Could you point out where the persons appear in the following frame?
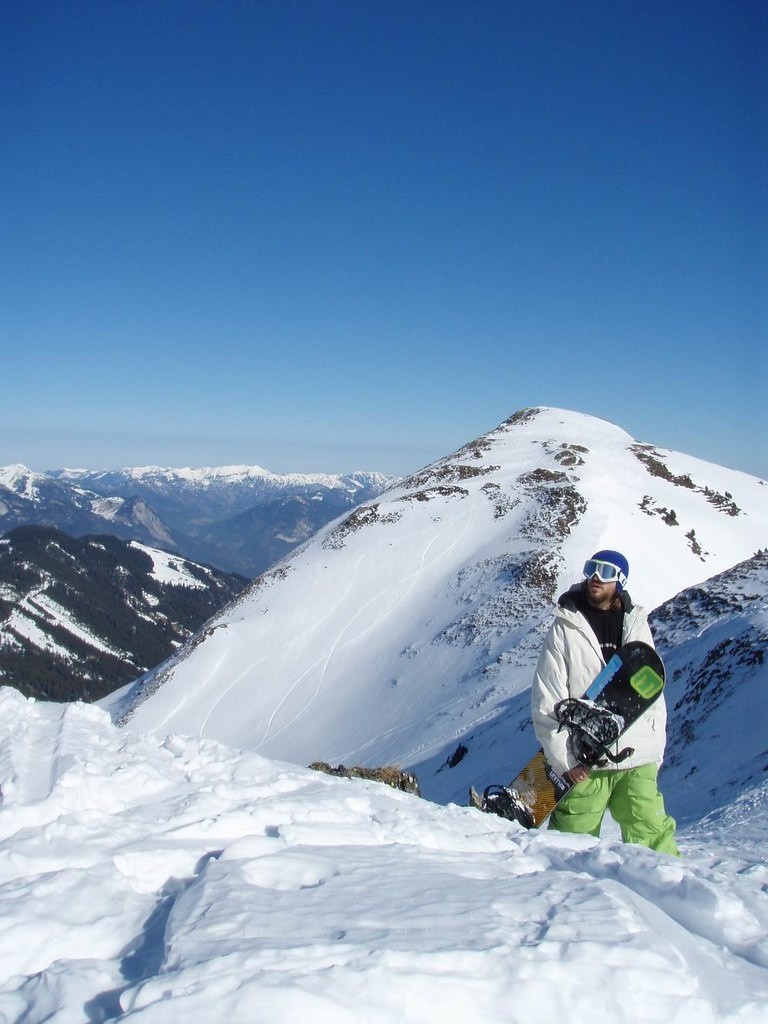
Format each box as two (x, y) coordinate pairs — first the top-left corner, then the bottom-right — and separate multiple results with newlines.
(530, 550), (681, 858)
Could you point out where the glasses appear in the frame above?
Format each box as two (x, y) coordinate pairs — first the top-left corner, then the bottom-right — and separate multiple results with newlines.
(582, 560), (620, 584)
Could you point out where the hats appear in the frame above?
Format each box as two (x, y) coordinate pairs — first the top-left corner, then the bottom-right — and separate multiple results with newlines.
(582, 549), (629, 594)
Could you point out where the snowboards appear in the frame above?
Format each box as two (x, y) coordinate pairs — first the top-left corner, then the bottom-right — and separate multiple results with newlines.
(485, 641), (666, 827)
